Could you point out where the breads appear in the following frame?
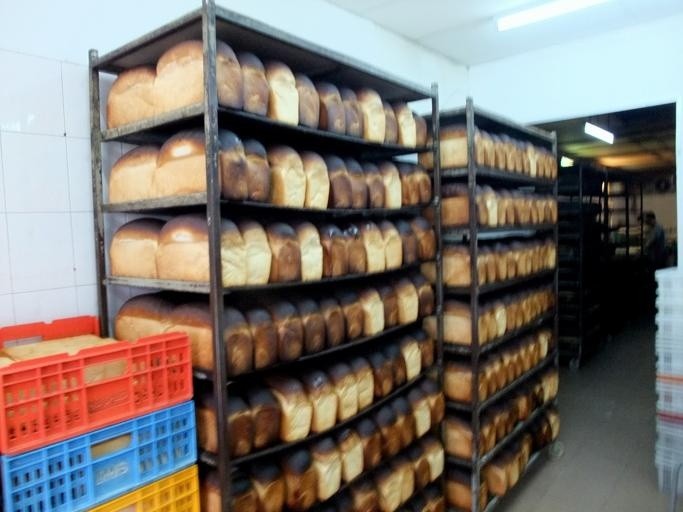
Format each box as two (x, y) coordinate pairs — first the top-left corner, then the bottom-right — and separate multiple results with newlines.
(0, 39), (561, 511)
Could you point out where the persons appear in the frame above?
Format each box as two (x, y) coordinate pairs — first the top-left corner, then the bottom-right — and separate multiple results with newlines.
(636, 208), (665, 261)
